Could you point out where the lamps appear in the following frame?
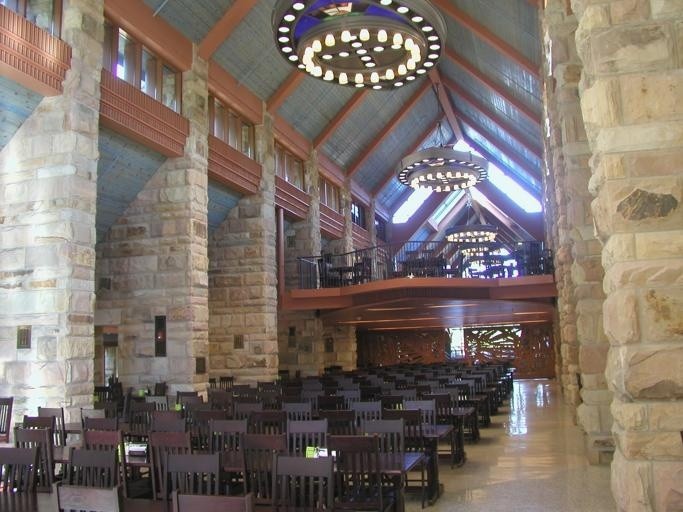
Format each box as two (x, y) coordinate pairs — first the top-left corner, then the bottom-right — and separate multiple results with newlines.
(447, 226), (496, 246)
(272, 1), (445, 93)
(393, 147), (488, 194)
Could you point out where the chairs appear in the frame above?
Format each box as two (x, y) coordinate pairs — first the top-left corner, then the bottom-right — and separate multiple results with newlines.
(319, 244), (550, 288)
